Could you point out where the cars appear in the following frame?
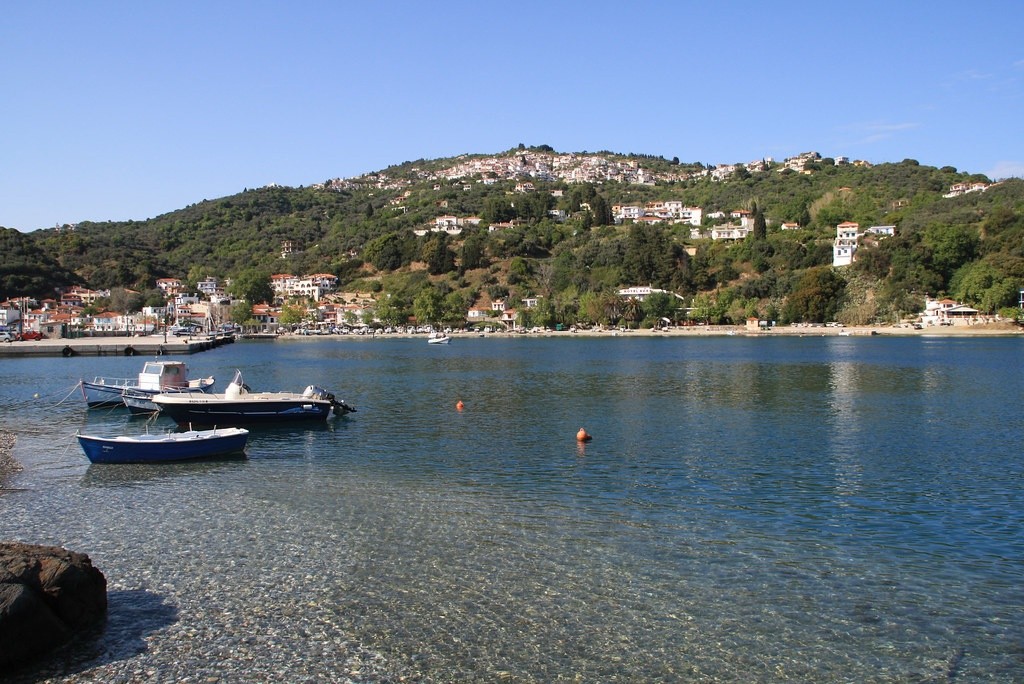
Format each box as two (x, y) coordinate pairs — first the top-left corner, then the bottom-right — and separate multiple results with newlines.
(16, 330), (45, 341)
(0, 332), (16, 343)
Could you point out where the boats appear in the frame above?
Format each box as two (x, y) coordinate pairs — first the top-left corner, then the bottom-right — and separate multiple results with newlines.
(150, 367), (358, 428)
(76, 428), (249, 464)
(427, 333), (450, 344)
(120, 359), (216, 416)
(79, 374), (141, 411)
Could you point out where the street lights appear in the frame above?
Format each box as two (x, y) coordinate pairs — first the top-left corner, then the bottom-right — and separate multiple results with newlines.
(164, 294), (168, 343)
